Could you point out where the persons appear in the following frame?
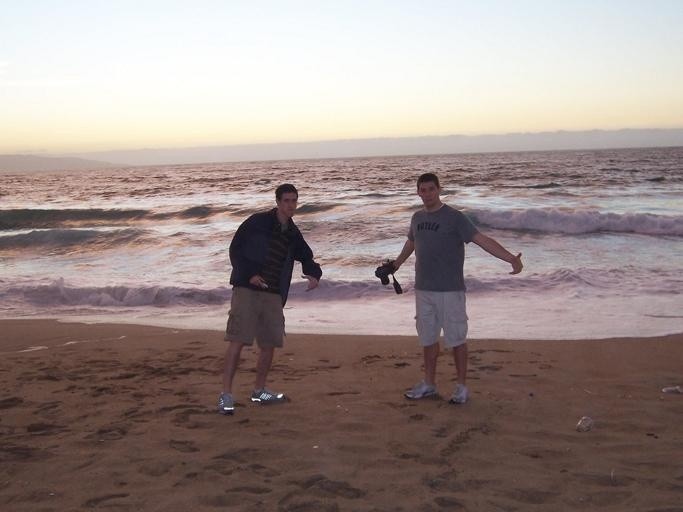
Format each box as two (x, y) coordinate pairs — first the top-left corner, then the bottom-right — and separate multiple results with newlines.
(218, 183), (322, 415)
(376, 173), (523, 404)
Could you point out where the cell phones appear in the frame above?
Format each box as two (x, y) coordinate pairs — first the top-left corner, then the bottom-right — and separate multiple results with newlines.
(263, 282), (269, 289)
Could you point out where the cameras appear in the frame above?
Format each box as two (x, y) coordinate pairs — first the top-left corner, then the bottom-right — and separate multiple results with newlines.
(375, 264), (396, 285)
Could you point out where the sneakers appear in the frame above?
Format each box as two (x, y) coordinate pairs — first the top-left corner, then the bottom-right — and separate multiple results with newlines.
(448, 383), (467, 403)
(251, 387), (284, 401)
(217, 392), (234, 414)
(404, 380), (437, 398)
